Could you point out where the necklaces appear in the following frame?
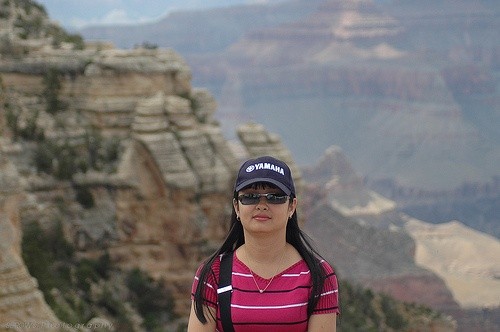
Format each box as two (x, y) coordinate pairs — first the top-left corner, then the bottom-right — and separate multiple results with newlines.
(245, 249), (283, 293)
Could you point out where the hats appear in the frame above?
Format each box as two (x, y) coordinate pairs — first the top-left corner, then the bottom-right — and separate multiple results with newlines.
(236, 156), (295, 195)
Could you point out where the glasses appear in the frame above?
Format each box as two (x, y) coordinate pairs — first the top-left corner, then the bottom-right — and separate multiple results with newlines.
(239, 192), (287, 204)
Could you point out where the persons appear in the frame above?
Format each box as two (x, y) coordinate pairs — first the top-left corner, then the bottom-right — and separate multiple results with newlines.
(186, 157), (342, 332)
